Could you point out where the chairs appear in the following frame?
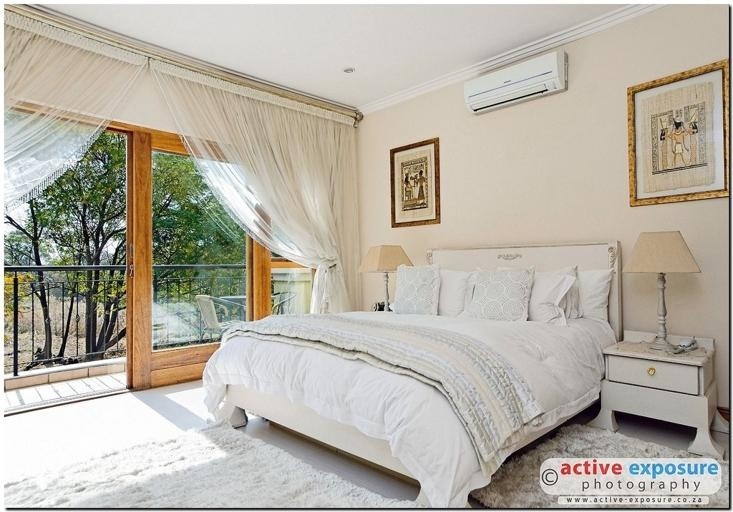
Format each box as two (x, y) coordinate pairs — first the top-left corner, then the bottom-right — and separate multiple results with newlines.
(195, 294), (247, 340)
(270, 290), (300, 315)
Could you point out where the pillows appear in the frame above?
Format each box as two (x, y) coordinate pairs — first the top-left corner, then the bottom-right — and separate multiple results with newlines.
(389, 263), (614, 326)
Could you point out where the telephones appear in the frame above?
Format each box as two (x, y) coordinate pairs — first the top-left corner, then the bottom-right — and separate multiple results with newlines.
(679, 338), (697, 351)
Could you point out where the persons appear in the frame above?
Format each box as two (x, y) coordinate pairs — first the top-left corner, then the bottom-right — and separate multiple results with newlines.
(660, 118), (698, 169)
(403, 170), (425, 207)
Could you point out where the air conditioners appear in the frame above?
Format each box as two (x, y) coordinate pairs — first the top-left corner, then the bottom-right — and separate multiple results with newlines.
(461, 50), (570, 116)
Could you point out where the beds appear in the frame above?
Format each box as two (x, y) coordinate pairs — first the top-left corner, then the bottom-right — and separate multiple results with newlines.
(197, 238), (628, 509)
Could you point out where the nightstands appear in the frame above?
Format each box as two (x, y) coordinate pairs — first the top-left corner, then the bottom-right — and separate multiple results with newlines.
(584, 328), (730, 460)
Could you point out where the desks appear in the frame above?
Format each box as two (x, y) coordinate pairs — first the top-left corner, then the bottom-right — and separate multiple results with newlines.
(219, 294), (277, 322)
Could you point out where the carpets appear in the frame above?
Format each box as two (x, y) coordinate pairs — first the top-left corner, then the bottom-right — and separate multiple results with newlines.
(5, 411), (428, 508)
(470, 419), (728, 510)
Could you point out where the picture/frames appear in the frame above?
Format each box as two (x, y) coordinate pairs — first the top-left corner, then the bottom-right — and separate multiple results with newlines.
(623, 58), (729, 209)
(389, 135), (440, 230)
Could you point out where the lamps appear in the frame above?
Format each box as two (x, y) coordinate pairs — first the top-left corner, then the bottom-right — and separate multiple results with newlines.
(620, 229), (701, 353)
(355, 243), (413, 312)
